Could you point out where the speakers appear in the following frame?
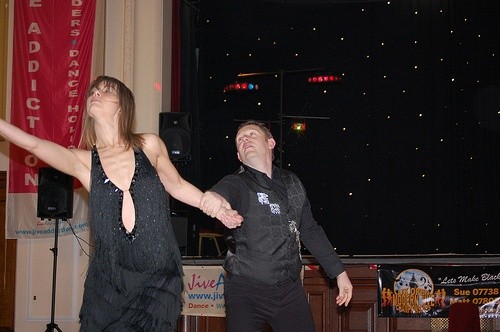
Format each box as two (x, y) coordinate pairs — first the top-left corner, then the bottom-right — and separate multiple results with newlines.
(37, 167), (73, 221)
(159, 112), (193, 165)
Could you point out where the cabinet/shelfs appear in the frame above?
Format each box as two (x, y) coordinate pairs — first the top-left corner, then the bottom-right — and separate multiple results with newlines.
(184, 264), (431, 332)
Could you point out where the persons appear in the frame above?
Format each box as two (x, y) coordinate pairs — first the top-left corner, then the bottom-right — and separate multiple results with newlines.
(0, 75), (244, 332)
(200, 120), (353, 332)
